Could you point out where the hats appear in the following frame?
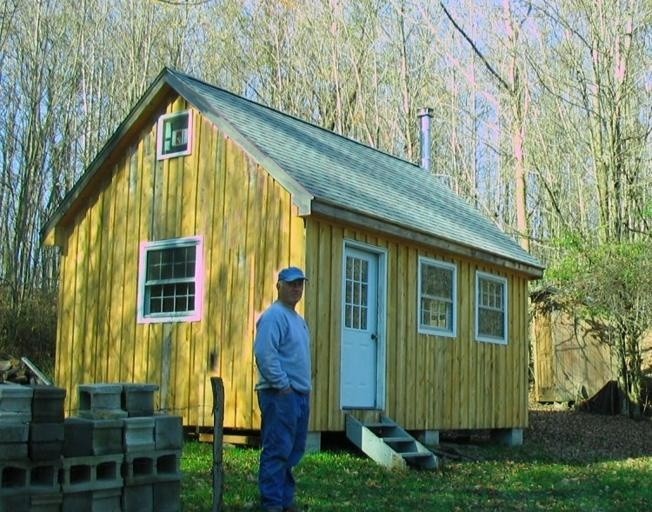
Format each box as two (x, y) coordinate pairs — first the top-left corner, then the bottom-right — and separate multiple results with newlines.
(277, 267), (309, 284)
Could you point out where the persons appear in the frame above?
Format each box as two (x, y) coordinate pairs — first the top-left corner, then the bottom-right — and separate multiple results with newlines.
(250, 266), (313, 509)
